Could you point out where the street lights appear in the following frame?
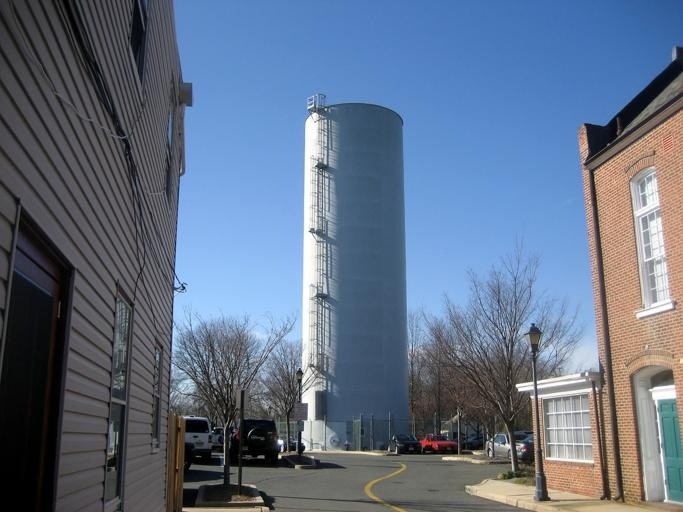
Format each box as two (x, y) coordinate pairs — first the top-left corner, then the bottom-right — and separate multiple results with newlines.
(524, 322), (553, 502)
(454, 399), (465, 455)
(296, 367), (305, 456)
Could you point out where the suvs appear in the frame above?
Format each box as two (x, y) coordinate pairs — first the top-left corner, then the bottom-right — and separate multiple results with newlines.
(230, 417), (278, 465)
(211, 426), (228, 452)
(183, 415), (213, 464)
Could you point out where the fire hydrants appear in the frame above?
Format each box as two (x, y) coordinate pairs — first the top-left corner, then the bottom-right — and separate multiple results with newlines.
(344, 441), (351, 451)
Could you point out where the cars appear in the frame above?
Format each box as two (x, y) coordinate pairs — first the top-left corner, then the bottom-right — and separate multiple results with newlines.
(277, 434), (305, 453)
(385, 433), (421, 455)
(419, 433), (458, 453)
(463, 432), (491, 450)
(486, 431), (528, 461)
